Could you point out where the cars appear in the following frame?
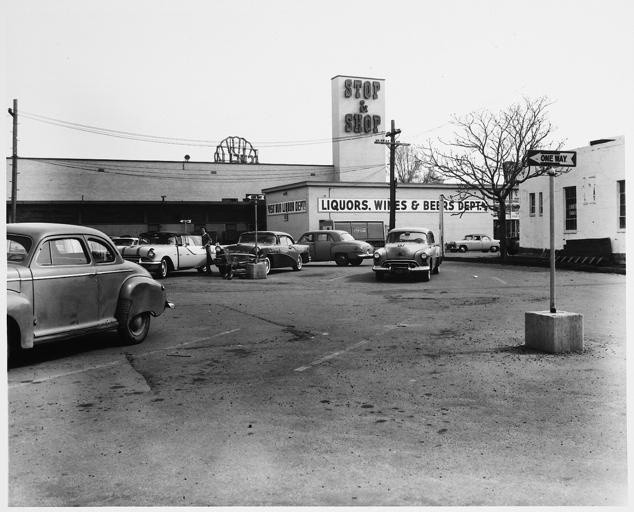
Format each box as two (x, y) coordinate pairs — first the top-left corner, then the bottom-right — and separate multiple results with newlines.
(372, 227), (441, 279)
(213, 231), (312, 276)
(108, 231), (231, 279)
(294, 230), (375, 267)
(7, 219), (176, 367)
(446, 234), (503, 254)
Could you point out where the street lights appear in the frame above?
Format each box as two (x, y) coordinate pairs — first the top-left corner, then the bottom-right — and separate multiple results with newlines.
(247, 194), (265, 264)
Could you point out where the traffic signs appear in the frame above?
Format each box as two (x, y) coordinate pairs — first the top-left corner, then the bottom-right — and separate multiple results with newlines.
(527, 151), (579, 168)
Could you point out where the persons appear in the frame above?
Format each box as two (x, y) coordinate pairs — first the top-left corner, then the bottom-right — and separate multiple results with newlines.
(215, 245), (234, 279)
(200, 227), (213, 274)
